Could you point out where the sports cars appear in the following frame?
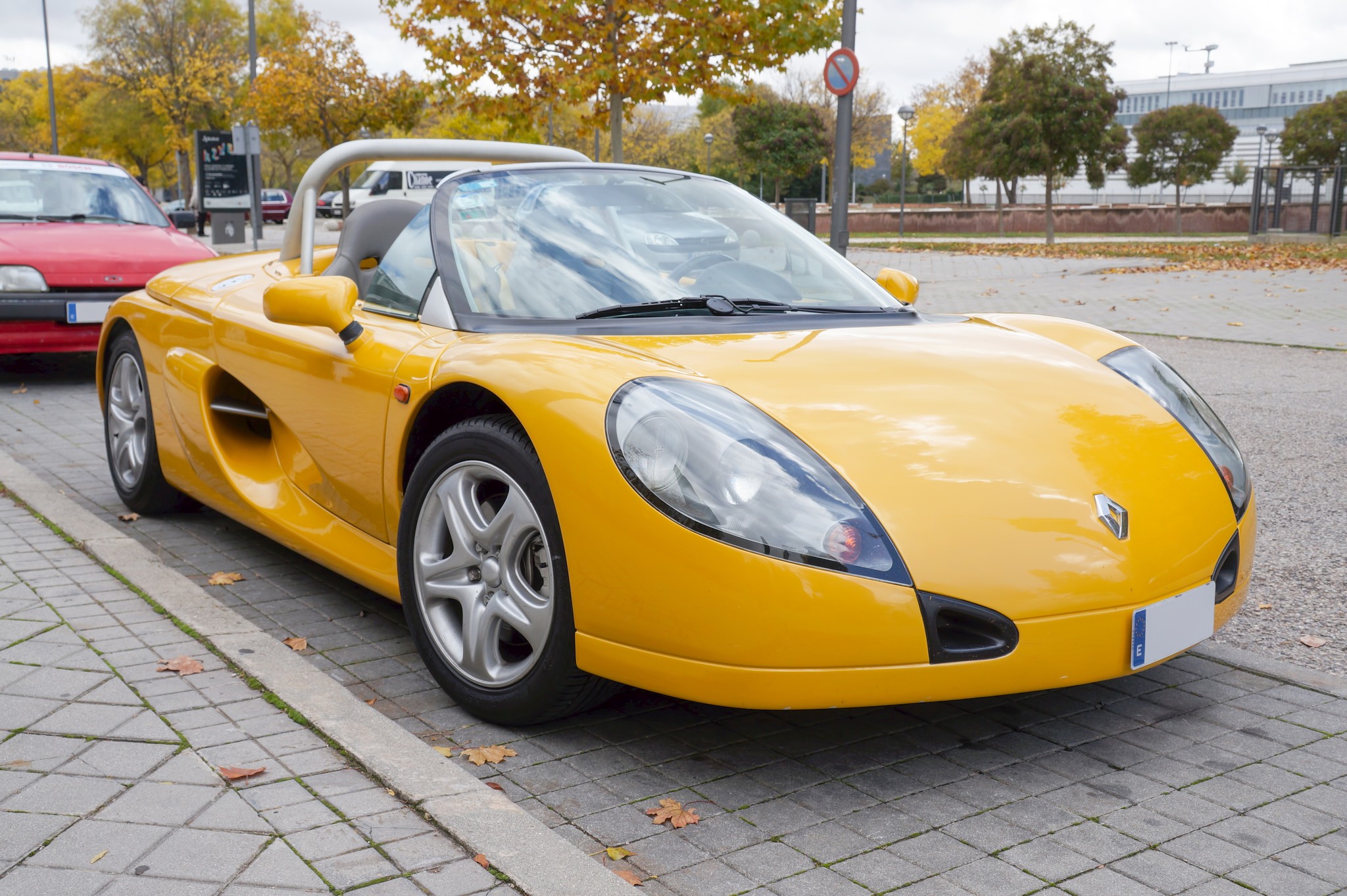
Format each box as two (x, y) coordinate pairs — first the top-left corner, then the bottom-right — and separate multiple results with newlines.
(96, 138), (1257, 726)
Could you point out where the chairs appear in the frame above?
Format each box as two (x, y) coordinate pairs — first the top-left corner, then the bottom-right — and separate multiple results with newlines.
(509, 186), (633, 319)
(321, 197), (510, 319)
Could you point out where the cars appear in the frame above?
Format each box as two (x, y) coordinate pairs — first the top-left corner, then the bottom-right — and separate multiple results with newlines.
(316, 190), (344, 217)
(0, 150), (221, 362)
(207, 187), (294, 225)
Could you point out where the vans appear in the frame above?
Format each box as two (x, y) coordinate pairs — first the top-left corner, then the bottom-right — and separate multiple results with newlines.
(332, 161), (491, 218)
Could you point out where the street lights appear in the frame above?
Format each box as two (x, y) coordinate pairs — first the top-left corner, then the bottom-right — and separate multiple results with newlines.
(1264, 130), (1281, 207)
(703, 133), (714, 175)
(1257, 124), (1268, 204)
(898, 103), (914, 235)
